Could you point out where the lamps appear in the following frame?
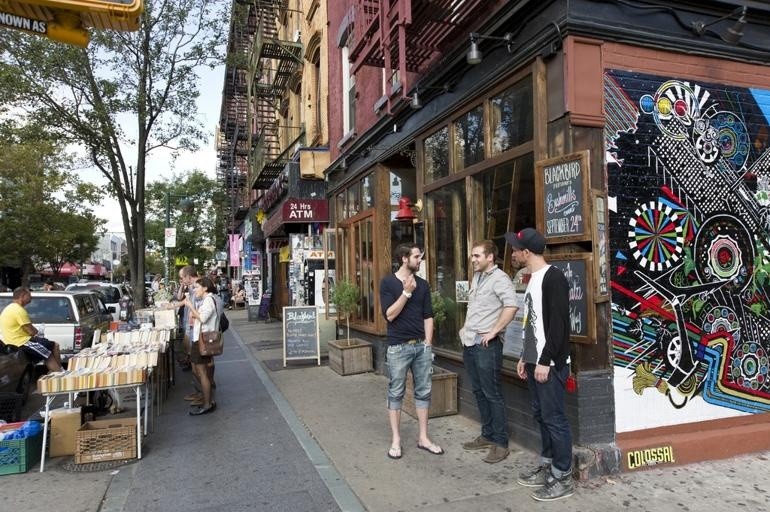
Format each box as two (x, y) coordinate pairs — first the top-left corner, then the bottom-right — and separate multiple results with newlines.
(690, 3), (750, 47)
(322, 169), (338, 183)
(338, 151), (365, 169)
(465, 31), (518, 66)
(407, 78), (456, 111)
(394, 196), (424, 219)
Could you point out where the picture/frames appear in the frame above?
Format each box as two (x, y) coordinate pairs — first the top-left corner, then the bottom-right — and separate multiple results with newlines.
(587, 188), (610, 304)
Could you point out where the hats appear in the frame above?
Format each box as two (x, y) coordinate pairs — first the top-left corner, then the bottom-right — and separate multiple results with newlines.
(503, 227), (546, 255)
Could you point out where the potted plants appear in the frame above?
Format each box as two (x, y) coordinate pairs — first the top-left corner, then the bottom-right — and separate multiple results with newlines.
(325, 277), (375, 376)
(401, 281), (460, 421)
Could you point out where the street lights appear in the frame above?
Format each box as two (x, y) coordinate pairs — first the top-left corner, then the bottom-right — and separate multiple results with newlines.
(162, 190), (198, 294)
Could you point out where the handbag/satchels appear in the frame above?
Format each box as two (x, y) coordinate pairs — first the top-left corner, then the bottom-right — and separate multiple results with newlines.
(198, 330), (225, 358)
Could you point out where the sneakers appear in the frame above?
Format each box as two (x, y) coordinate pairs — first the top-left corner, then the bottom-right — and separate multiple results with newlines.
(182, 392), (198, 401)
(482, 445), (511, 464)
(186, 403), (214, 416)
(514, 460), (553, 488)
(462, 434), (494, 450)
(188, 394), (205, 406)
(529, 465), (576, 502)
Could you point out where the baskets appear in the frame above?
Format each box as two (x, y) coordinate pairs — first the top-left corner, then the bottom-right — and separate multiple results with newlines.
(73, 417), (144, 464)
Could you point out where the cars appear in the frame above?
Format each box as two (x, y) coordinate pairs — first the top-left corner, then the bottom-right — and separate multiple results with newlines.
(0, 280), (153, 377)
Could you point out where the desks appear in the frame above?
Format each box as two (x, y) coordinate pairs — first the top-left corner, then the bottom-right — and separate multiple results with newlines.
(36, 331), (175, 474)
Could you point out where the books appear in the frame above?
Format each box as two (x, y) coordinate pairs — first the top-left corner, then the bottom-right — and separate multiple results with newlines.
(35, 304), (177, 394)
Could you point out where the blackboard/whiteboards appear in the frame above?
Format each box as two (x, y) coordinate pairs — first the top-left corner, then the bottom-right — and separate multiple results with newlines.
(283, 305), (321, 359)
(589, 188), (613, 304)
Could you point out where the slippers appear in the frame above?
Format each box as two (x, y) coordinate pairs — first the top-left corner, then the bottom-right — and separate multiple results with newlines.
(387, 442), (404, 460)
(415, 440), (444, 456)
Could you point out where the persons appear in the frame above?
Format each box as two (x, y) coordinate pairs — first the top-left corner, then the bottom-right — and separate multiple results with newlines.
(503, 228), (577, 503)
(0, 285), (61, 372)
(458, 239), (520, 462)
(380, 242), (443, 459)
(173, 264), (216, 406)
(184, 277), (223, 416)
(151, 274), (162, 292)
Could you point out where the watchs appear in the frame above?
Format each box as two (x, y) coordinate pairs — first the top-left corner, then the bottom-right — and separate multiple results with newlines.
(402, 290), (412, 298)
